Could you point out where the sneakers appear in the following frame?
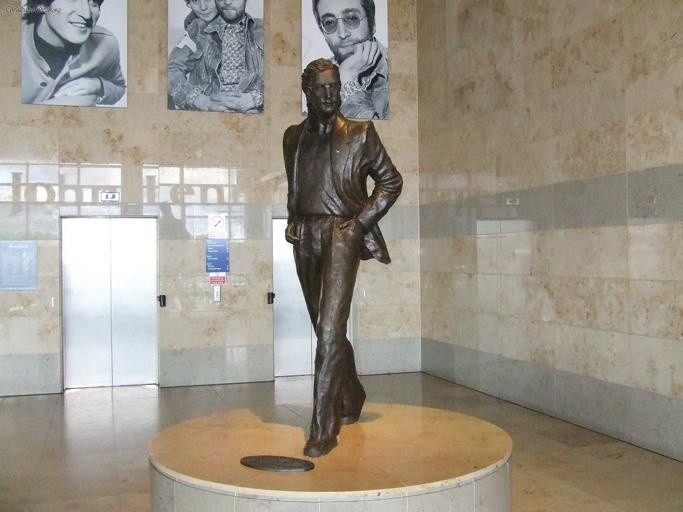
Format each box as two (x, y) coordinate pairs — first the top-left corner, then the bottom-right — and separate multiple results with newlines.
(302, 436), (338, 459)
(343, 386), (366, 426)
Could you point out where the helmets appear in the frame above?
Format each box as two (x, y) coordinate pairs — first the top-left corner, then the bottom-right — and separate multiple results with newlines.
(320, 15), (361, 34)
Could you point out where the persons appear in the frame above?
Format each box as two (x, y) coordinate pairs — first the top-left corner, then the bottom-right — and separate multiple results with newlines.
(167, 0), (264, 113)
(282, 57), (404, 454)
(183, 0), (219, 30)
(301, 0), (389, 118)
(19, 0), (127, 106)
